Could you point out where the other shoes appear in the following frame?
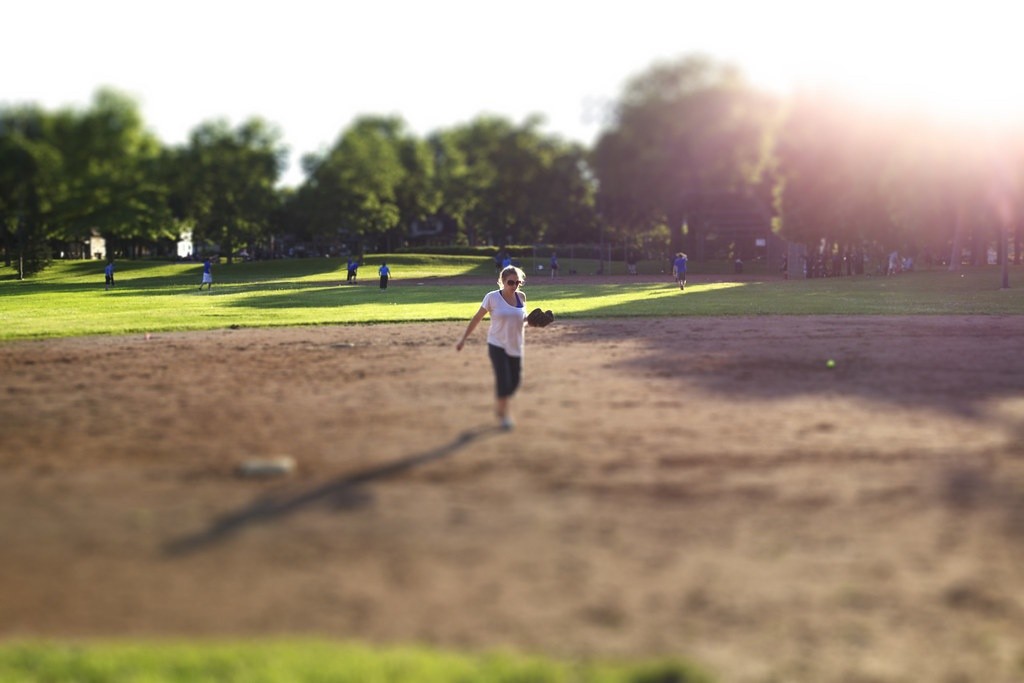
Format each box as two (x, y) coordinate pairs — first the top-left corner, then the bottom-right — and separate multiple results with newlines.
(500, 418), (513, 428)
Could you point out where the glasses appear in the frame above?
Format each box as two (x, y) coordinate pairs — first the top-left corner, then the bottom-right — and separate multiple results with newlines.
(506, 280), (521, 286)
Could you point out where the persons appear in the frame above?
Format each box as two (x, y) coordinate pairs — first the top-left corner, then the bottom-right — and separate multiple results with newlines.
(673, 252), (687, 291)
(199, 256), (212, 291)
(456, 265), (554, 429)
(104, 258), (115, 291)
(379, 261), (391, 290)
(492, 251), (561, 283)
(626, 250), (637, 275)
(345, 254), (360, 285)
(781, 240), (916, 279)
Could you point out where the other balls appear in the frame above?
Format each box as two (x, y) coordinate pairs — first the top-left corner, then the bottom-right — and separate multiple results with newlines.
(826, 358), (837, 370)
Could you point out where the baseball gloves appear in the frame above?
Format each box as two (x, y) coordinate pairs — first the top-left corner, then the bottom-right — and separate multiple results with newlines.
(527, 307), (553, 328)
